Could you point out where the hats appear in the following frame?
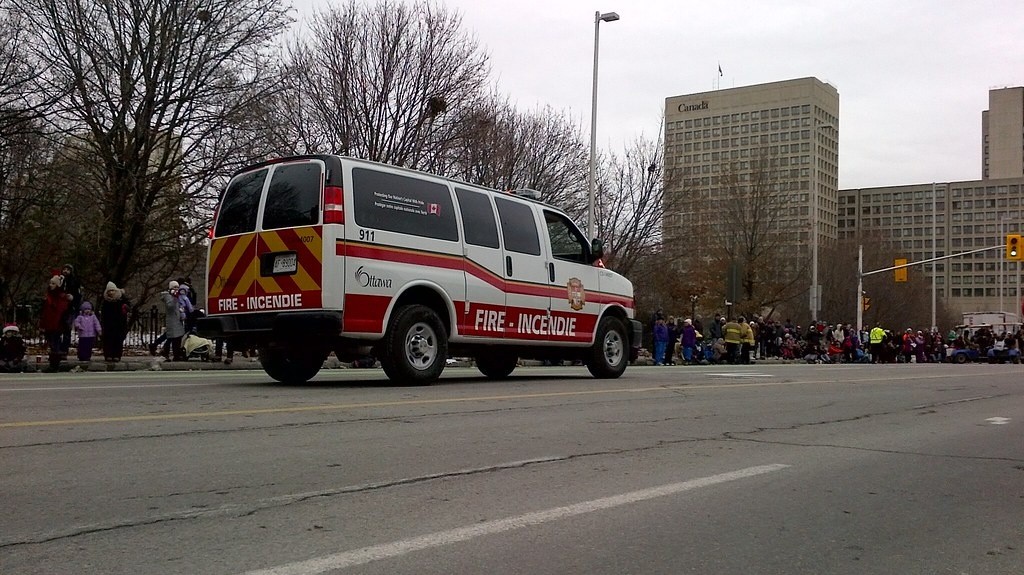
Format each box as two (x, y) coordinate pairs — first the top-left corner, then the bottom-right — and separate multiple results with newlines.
(51, 275), (65, 287)
(684, 319), (692, 325)
(104, 281), (118, 295)
(81, 302), (92, 311)
(169, 281), (179, 289)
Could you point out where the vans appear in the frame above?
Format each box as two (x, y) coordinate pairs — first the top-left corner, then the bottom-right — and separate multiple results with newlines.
(196, 152), (643, 387)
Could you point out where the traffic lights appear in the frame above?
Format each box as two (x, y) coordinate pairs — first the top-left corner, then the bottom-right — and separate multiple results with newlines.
(864, 298), (870, 311)
(1006, 235), (1022, 260)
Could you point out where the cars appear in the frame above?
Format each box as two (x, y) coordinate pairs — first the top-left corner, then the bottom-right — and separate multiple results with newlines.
(944, 329), (1023, 365)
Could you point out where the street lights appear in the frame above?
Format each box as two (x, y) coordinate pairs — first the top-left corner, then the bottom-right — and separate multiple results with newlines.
(587, 10), (621, 244)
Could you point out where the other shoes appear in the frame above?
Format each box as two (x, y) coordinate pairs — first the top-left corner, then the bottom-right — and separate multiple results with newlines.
(224, 358), (233, 365)
(671, 363), (674, 366)
(148, 343), (157, 356)
(666, 363), (669, 365)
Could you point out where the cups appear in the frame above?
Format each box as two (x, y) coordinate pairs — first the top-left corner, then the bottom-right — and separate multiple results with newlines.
(36, 355), (41, 363)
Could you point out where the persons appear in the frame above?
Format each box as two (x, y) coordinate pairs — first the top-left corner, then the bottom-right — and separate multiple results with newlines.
(40, 263), (80, 366)
(148, 276), (198, 362)
(648, 308), (1024, 366)
(72, 302), (103, 363)
(0, 322), (26, 368)
(99, 280), (132, 363)
(211, 338), (235, 365)
(240, 342), (260, 357)
